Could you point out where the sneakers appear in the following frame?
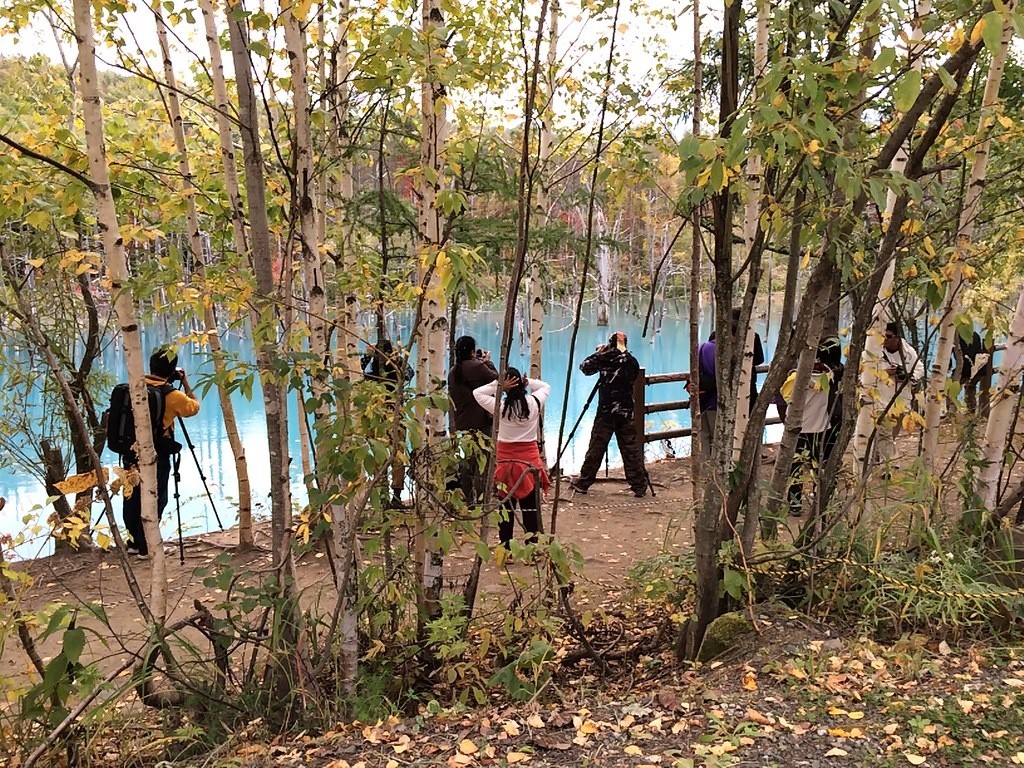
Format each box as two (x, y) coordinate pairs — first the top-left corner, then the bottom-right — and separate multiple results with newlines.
(893, 463), (901, 470)
(570, 480), (589, 495)
(879, 467), (891, 481)
(504, 553), (514, 565)
(789, 506), (801, 516)
(631, 487), (647, 497)
(126, 545), (149, 559)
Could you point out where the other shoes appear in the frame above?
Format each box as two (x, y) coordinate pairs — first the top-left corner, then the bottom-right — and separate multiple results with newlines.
(381, 500), (411, 510)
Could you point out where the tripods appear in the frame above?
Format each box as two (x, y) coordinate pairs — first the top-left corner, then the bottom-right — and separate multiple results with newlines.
(549, 378), (656, 496)
(90, 416), (225, 565)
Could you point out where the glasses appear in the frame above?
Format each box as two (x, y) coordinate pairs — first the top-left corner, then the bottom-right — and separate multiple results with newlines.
(884, 334), (897, 339)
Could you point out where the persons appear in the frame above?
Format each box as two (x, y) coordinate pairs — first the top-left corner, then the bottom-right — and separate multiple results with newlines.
(122, 348), (200, 560)
(570, 332), (647, 498)
(698, 307), (764, 483)
(776, 328), (844, 517)
(961, 331), (980, 416)
(448, 336), (499, 511)
(877, 322), (925, 480)
(473, 367), (551, 565)
(361, 339), (414, 511)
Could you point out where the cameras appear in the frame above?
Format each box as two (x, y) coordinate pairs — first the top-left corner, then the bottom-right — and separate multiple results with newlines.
(476, 350), (487, 361)
(168, 368), (182, 383)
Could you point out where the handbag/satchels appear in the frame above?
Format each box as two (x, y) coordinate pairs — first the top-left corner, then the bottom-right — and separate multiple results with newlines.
(537, 440), (544, 454)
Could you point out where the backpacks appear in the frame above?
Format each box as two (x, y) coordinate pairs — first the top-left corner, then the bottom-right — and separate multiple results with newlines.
(108, 383), (166, 455)
